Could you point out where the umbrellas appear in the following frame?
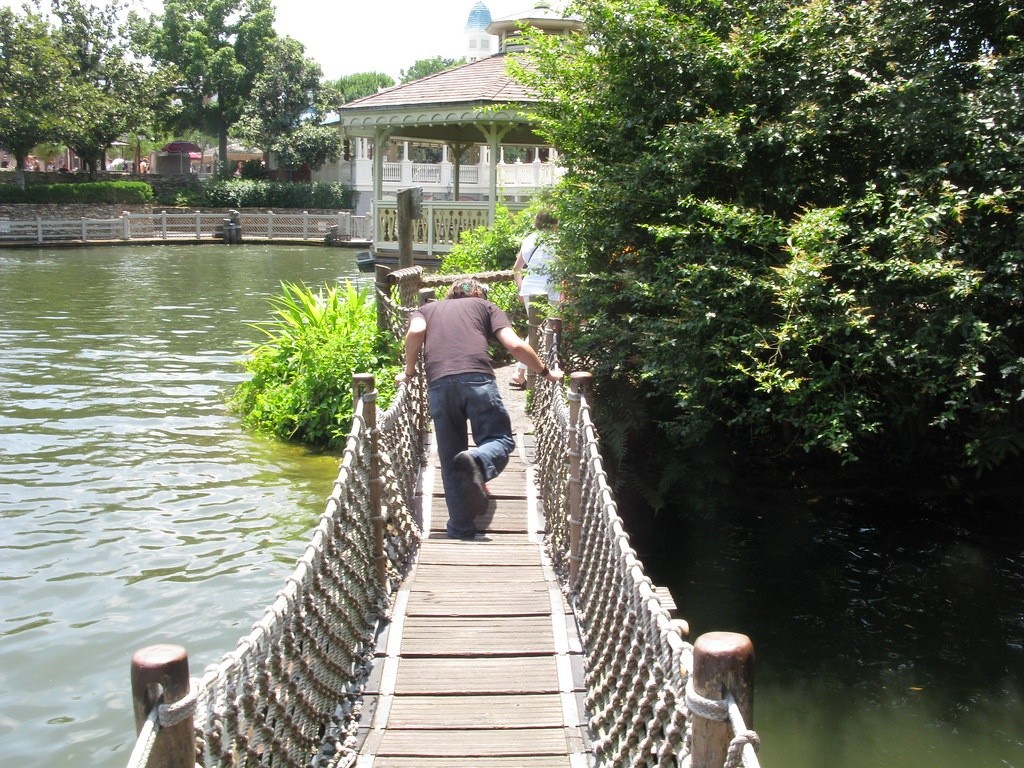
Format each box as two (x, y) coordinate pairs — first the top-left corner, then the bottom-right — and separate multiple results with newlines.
(162, 140), (202, 174)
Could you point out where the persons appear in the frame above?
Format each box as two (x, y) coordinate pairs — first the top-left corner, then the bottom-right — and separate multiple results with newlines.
(510, 208), (566, 391)
(1, 156), (149, 175)
(393, 275), (567, 540)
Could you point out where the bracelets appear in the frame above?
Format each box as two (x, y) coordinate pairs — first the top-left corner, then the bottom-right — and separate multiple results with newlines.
(405, 370), (416, 378)
(536, 366), (550, 380)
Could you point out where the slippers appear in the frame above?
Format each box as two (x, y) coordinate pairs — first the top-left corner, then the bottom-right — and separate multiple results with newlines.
(509, 380), (528, 390)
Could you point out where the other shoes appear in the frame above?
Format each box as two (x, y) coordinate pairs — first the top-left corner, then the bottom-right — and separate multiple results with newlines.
(454, 451), (487, 515)
(448, 526), (476, 540)
